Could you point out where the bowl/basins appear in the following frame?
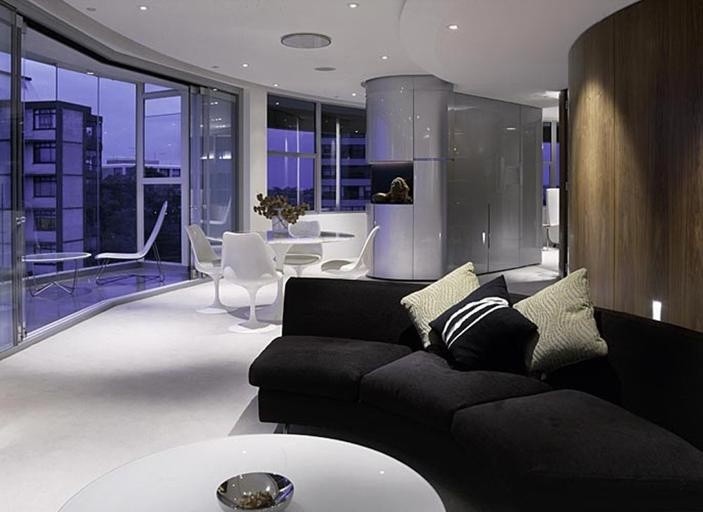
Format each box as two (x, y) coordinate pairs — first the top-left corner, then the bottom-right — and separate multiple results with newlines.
(216, 472), (293, 511)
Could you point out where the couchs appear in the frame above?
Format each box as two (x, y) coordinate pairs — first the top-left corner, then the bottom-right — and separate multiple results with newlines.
(246, 278), (703, 512)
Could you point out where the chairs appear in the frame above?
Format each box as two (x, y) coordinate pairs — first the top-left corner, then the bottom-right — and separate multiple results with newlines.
(217, 231), (281, 329)
(543, 188), (560, 246)
(92, 199), (170, 281)
(183, 222), (239, 315)
(320, 224), (381, 279)
(273, 221), (322, 276)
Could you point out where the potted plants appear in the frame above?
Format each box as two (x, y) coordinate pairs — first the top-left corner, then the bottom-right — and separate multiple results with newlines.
(253, 189), (309, 239)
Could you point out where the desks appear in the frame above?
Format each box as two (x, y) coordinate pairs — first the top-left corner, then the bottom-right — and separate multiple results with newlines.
(219, 229), (355, 321)
(21, 250), (92, 297)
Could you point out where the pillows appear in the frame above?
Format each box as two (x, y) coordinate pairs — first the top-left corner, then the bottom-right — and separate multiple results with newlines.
(399, 261), (481, 349)
(427, 273), (541, 371)
(510, 266), (609, 380)
